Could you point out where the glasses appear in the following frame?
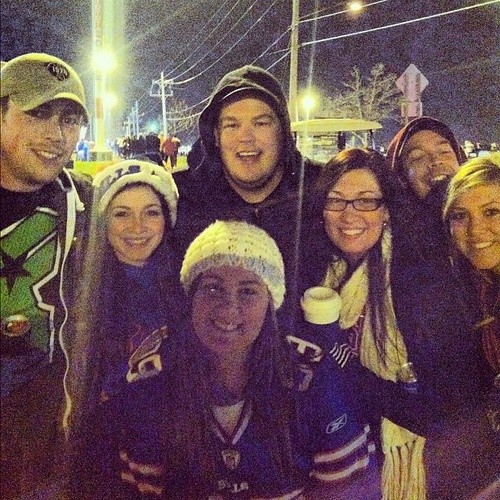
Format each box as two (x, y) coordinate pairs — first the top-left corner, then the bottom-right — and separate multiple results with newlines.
(324, 195), (386, 213)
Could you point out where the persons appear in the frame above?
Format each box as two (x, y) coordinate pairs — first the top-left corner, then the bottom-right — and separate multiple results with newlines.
(157, 64), (335, 338)
(114, 133), (181, 168)
(1, 51), (96, 493)
(282, 146), (481, 500)
(74, 155), (187, 417)
(385, 114), (468, 202)
(441, 152), (500, 498)
(82, 216), (381, 500)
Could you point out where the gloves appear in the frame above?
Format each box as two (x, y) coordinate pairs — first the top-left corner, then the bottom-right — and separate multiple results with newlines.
(298, 322), (353, 373)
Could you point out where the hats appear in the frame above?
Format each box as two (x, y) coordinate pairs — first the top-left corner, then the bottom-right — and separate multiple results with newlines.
(180, 221), (286, 309)
(91, 154), (180, 228)
(0, 51), (90, 125)
